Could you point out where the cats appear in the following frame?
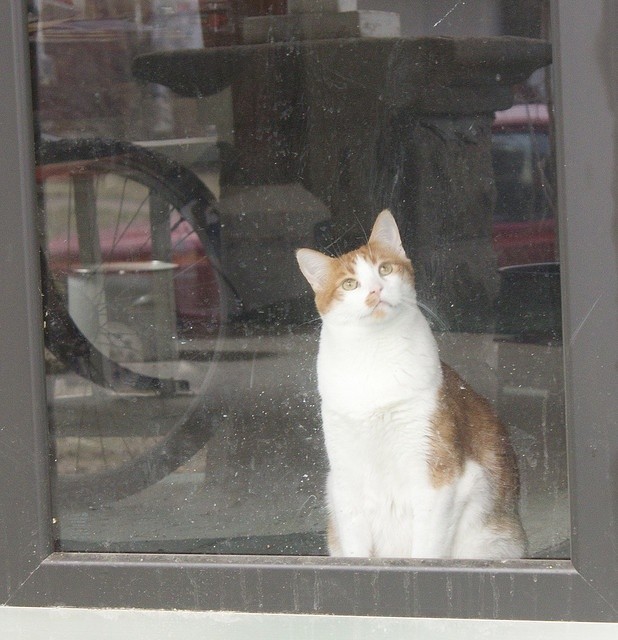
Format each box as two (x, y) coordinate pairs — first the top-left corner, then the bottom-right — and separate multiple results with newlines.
(297, 208), (528, 560)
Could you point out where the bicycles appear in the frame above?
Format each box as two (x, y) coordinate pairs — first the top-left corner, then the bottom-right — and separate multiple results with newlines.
(37, 137), (244, 507)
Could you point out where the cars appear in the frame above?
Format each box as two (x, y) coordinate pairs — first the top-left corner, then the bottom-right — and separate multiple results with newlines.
(44, 103), (562, 340)
